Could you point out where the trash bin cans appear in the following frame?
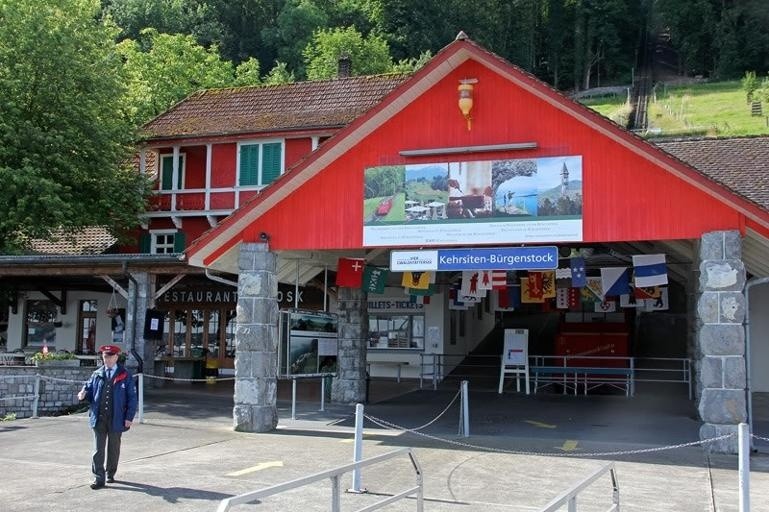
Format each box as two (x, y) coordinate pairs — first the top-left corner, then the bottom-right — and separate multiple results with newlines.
(206, 359), (218, 385)
(194, 360), (205, 384)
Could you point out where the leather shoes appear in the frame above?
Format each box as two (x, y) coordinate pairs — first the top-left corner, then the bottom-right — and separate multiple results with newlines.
(90, 484), (104, 489)
(106, 471), (114, 483)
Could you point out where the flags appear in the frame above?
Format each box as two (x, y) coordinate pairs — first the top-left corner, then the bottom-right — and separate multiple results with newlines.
(336, 253), (669, 313)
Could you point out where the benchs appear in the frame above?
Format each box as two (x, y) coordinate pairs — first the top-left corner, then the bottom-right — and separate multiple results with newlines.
(367, 360), (409, 384)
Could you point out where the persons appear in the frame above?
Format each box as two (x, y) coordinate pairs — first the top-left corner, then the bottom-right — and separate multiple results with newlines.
(76, 345), (138, 490)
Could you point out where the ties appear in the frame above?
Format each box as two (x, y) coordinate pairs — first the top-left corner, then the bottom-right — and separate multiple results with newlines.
(107, 369), (112, 378)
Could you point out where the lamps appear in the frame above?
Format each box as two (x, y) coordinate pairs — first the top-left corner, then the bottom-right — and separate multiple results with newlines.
(457, 79), (479, 132)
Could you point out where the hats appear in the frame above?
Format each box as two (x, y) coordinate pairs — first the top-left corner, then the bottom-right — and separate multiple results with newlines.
(99, 345), (122, 355)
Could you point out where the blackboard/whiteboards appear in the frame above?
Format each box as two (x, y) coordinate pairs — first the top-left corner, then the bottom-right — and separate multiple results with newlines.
(143, 308), (163, 340)
(505, 332), (526, 365)
(112, 308), (125, 333)
